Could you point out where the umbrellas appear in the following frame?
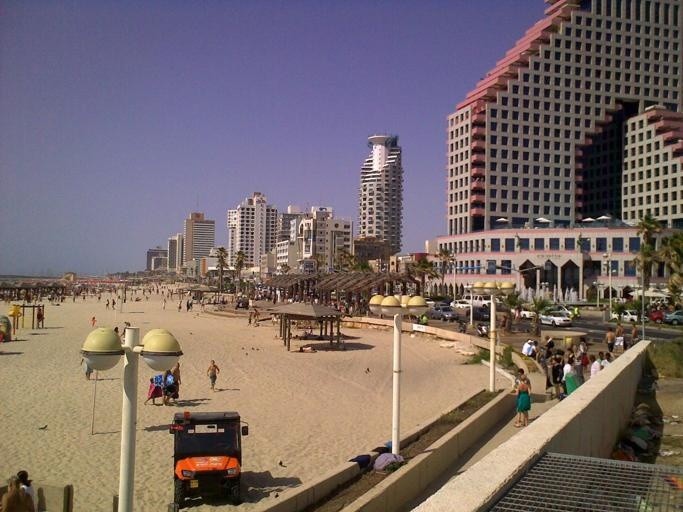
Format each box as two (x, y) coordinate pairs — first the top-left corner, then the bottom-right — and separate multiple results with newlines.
(496, 218), (509, 222)
(539, 219), (552, 223)
(535, 217), (547, 220)
(595, 216), (611, 220)
(581, 217), (595, 222)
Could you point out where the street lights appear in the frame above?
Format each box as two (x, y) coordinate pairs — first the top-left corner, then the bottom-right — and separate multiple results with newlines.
(369, 293), (429, 456)
(449, 260), (456, 308)
(79, 326), (184, 512)
(602, 250), (612, 321)
(472, 281), (516, 393)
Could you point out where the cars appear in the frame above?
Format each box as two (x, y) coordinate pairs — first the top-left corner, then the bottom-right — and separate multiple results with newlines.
(423, 293), (503, 321)
(611, 309), (683, 326)
(510, 304), (574, 328)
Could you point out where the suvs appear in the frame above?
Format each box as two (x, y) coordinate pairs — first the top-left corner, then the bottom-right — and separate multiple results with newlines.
(169, 411), (248, 509)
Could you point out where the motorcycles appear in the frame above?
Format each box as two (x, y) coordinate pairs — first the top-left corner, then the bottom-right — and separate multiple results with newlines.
(235, 302), (249, 310)
(456, 320), (468, 334)
(473, 323), (490, 338)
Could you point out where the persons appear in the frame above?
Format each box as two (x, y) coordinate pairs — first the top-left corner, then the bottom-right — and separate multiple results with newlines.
(641, 293), (683, 321)
(290, 346), (316, 353)
(507, 375), (531, 427)
(510, 368), (531, 425)
(80, 352), (92, 379)
(521, 317), (639, 397)
(15, 470), (33, 506)
(169, 362), (181, 403)
(206, 360), (219, 391)
(143, 378), (159, 405)
(160, 367), (177, 406)
(1, 279), (362, 341)
(410, 299), (524, 339)
(0, 476), (33, 511)
